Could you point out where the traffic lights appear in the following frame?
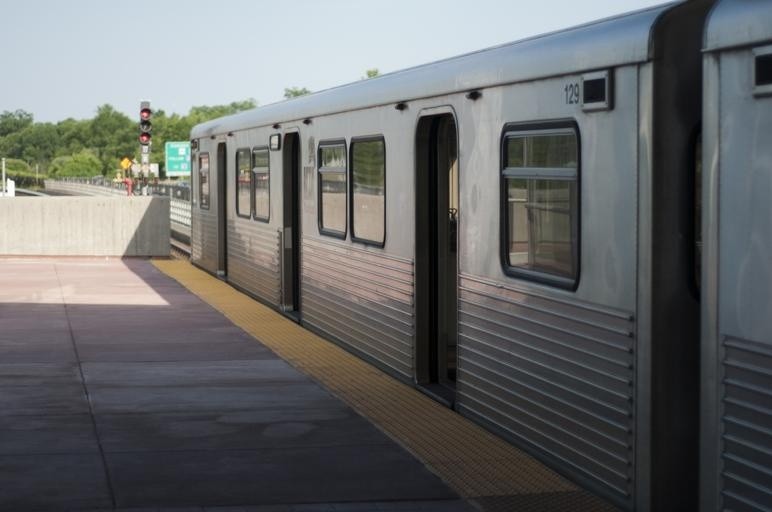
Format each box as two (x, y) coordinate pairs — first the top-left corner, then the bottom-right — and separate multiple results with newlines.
(140, 101), (151, 146)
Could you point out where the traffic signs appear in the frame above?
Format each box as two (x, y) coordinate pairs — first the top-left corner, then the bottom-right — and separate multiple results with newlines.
(165, 142), (190, 172)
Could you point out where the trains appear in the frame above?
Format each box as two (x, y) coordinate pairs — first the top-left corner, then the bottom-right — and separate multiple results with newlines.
(189, 2), (771, 510)
